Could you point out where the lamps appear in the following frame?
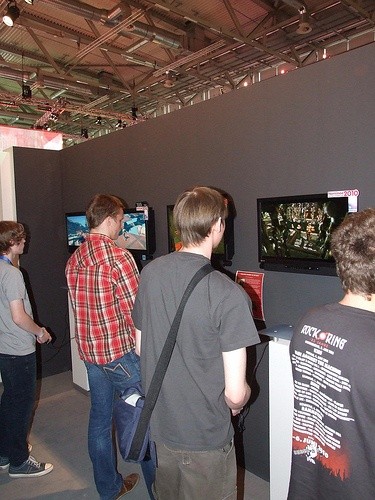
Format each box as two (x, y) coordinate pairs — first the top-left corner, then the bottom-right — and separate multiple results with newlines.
(2, 0), (20, 27)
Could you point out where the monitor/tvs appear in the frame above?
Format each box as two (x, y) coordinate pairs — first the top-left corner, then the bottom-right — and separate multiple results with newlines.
(67, 207), (150, 260)
(167, 205), (228, 261)
(257, 189), (359, 275)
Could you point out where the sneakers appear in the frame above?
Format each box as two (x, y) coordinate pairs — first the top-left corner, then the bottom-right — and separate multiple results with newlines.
(113, 472), (139, 499)
(7, 455), (55, 477)
(0, 442), (32, 469)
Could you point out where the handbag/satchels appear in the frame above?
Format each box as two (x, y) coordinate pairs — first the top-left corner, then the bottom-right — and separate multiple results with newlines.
(112, 387), (152, 464)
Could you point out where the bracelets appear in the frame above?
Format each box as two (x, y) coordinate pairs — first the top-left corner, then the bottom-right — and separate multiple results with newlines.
(37, 329), (44, 338)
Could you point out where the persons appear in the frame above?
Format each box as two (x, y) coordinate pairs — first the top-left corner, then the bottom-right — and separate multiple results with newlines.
(286, 209), (375, 499)
(64, 193), (157, 500)
(132, 187), (261, 500)
(0, 220), (54, 478)
